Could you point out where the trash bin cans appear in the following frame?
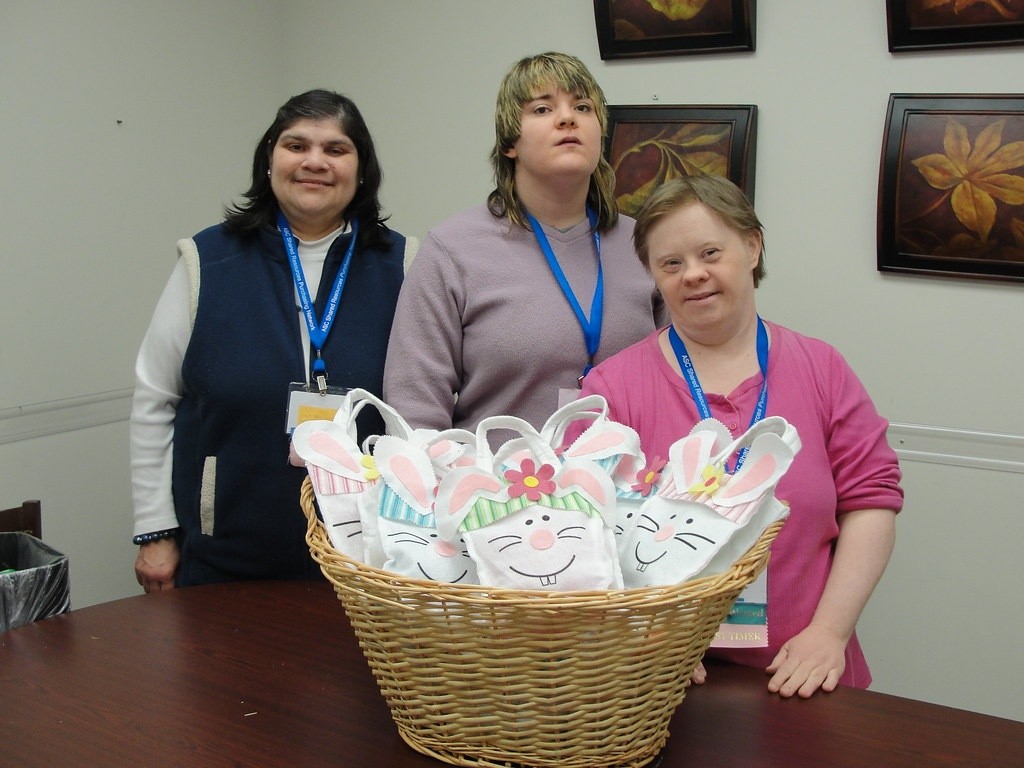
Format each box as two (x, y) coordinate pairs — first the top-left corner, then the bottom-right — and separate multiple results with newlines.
(0, 532), (72, 634)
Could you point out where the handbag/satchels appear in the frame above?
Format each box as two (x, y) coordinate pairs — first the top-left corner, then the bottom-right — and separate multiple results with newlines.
(306, 382), (804, 624)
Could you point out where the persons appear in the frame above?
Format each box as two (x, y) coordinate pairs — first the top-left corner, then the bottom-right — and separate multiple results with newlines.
(558, 174), (905, 698)
(381, 52), (672, 456)
(128, 89), (419, 597)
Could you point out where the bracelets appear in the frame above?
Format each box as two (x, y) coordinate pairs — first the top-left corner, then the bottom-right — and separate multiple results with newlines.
(133, 528), (176, 545)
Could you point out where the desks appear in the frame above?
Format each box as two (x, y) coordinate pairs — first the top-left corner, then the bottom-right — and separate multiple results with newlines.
(0, 579), (1024, 768)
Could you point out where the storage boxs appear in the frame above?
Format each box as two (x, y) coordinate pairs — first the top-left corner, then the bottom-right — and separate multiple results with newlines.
(0, 532), (71, 635)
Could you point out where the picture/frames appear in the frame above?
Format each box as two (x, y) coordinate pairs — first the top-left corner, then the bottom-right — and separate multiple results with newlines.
(602, 105), (758, 219)
(885, 0), (1024, 53)
(592, 0), (757, 60)
(876, 93), (1024, 283)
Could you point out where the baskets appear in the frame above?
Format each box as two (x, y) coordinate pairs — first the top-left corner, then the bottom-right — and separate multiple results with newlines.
(297, 454), (790, 768)
